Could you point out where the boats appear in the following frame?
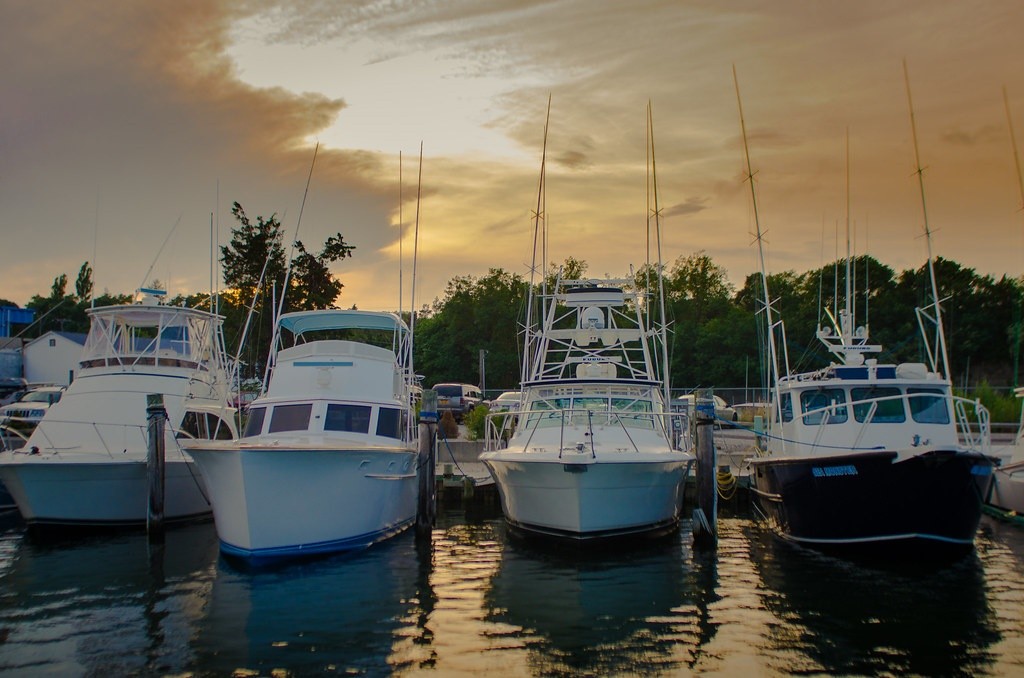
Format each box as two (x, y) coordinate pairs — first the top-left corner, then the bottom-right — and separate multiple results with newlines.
(476, 87), (696, 550)
(728, 55), (1000, 574)
(173, 141), (425, 572)
(0, 177), (240, 540)
(980, 389), (1024, 528)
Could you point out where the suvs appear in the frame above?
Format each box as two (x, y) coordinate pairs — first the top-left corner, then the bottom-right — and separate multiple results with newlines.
(430, 383), (485, 421)
(0, 378), (29, 396)
(0, 385), (65, 437)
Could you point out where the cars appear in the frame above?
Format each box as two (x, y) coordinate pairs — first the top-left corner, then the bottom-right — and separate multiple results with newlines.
(678, 394), (737, 430)
(488, 391), (527, 424)
